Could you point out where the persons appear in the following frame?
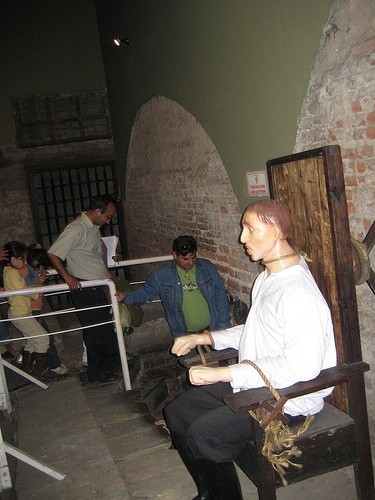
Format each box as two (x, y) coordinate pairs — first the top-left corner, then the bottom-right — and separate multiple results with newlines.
(46, 197), (144, 383)
(0, 240), (68, 375)
(107, 235), (233, 338)
(162, 198), (339, 500)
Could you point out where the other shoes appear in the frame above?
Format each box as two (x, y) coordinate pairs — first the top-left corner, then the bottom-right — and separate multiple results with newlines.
(51, 365), (68, 374)
(89, 374), (116, 382)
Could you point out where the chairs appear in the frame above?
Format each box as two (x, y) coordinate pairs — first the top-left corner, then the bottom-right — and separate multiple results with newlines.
(178, 145), (375, 500)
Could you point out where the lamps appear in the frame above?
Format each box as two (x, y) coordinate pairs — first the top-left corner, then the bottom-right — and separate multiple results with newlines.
(113, 37), (129, 47)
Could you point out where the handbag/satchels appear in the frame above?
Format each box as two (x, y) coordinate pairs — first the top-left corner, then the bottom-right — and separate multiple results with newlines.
(111, 303), (134, 335)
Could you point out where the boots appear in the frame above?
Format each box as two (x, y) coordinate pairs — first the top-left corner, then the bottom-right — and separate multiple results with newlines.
(31, 352), (55, 377)
(20, 351), (34, 373)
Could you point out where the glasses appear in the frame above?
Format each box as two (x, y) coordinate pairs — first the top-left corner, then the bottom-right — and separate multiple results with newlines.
(103, 210), (112, 222)
